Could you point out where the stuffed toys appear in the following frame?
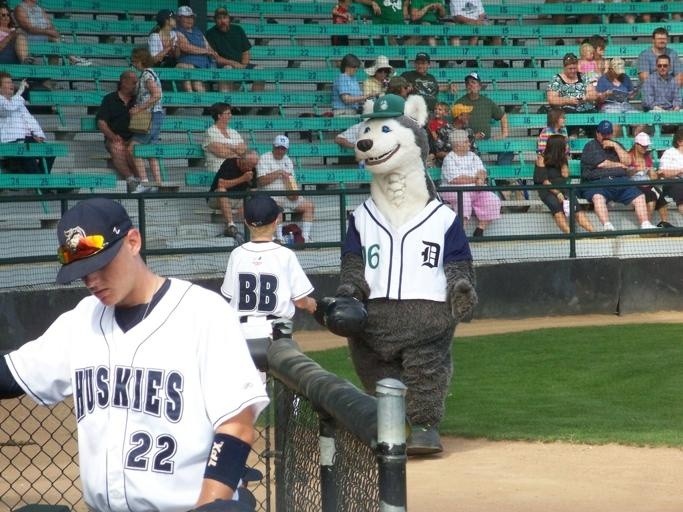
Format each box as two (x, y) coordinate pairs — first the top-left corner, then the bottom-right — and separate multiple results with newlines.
(314, 92), (479, 455)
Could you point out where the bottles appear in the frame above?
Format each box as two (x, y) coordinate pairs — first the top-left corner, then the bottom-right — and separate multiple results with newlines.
(288, 231), (294, 244)
(358, 159), (366, 181)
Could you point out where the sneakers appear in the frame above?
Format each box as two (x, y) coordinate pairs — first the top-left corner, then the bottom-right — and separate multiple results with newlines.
(640, 223), (656, 230)
(602, 221), (615, 231)
(130, 184), (152, 195)
(276, 237), (287, 245)
(223, 224), (240, 236)
(658, 223), (670, 228)
(127, 178), (137, 193)
(302, 233), (314, 243)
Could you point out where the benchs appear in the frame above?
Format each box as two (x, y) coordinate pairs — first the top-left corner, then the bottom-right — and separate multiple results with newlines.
(0, 0), (683, 225)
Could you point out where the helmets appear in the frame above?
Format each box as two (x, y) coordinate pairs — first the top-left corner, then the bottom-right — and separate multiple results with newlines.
(312, 296), (368, 337)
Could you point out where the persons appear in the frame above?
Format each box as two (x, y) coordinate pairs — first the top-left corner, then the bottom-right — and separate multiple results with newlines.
(0, 70), (55, 173)
(0, 3), (35, 65)
(220, 195), (318, 417)
(147, 5), (265, 91)
(127, 47), (162, 193)
(202, 102), (315, 244)
(14, 0), (90, 66)
(94, 70), (159, 193)
(0, 196), (271, 512)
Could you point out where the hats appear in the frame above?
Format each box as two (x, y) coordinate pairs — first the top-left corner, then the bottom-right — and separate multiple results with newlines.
(610, 57), (626, 75)
(363, 55), (394, 76)
(634, 131), (652, 147)
(272, 134), (290, 149)
(244, 196), (284, 227)
(464, 72), (481, 82)
(55, 198), (134, 284)
(177, 5), (197, 19)
(414, 52), (431, 63)
(597, 120), (614, 135)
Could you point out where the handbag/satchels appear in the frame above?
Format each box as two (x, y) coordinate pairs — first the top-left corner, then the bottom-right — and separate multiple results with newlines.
(127, 109), (155, 136)
